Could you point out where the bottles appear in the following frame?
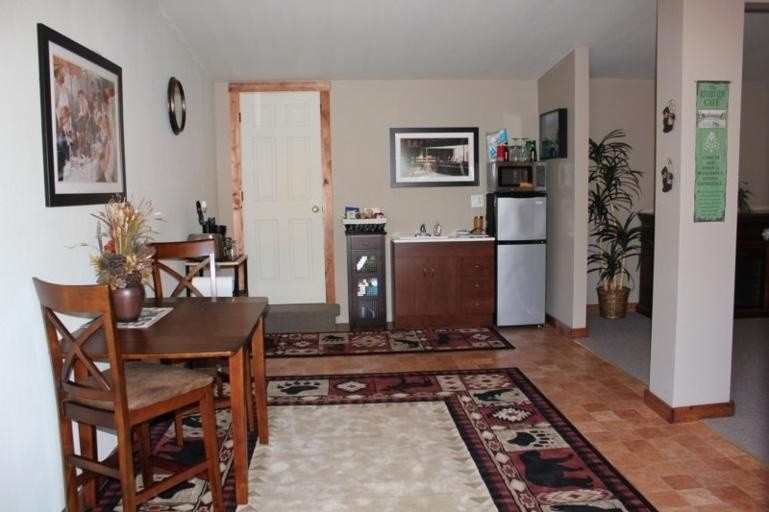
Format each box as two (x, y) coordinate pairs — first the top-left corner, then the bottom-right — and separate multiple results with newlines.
(473, 215), (484, 234)
(509, 137), (537, 161)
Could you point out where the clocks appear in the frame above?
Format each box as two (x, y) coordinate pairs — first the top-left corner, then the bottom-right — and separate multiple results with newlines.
(166, 76), (188, 135)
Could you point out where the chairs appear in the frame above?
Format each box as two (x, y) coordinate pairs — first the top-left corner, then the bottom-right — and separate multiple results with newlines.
(146, 238), (270, 454)
(34, 275), (230, 510)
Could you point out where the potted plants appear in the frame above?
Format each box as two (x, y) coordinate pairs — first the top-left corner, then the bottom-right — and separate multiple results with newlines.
(588, 128), (646, 321)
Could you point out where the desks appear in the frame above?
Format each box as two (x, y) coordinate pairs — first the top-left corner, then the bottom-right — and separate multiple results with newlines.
(636, 208), (768, 321)
(183, 253), (250, 297)
(56, 293), (267, 507)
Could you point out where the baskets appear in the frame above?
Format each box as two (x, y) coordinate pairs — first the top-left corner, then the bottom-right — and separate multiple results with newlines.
(342, 218), (387, 235)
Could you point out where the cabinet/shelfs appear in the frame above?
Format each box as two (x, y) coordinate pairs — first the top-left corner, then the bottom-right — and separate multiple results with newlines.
(344, 232), (496, 333)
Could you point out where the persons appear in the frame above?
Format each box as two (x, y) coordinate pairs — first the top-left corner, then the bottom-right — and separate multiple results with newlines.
(54, 64), (119, 183)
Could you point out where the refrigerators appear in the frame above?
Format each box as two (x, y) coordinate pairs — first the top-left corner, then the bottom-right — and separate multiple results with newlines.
(486, 191), (548, 328)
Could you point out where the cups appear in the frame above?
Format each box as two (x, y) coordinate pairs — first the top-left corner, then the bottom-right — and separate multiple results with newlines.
(497, 144), (509, 161)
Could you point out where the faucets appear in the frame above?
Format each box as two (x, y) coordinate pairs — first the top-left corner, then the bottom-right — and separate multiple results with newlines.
(420, 223), (426, 234)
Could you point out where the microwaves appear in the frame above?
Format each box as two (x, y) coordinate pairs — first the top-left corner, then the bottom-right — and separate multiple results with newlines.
(486, 161), (547, 194)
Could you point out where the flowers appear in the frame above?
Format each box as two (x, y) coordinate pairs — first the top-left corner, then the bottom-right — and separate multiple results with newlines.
(69, 194), (162, 289)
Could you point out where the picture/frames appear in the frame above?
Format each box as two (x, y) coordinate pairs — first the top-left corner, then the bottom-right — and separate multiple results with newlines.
(389, 126), (480, 187)
(36, 22), (128, 210)
(538, 108), (567, 160)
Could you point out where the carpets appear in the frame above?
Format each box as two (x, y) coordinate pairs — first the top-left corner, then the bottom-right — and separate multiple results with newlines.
(264, 328), (516, 359)
(63, 365), (658, 512)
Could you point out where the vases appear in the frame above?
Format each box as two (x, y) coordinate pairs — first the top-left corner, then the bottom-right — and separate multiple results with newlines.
(106, 287), (147, 325)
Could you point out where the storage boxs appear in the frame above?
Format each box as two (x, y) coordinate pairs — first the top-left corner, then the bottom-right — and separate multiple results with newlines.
(342, 217), (388, 231)
